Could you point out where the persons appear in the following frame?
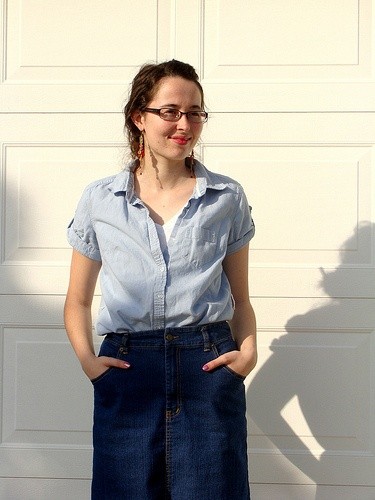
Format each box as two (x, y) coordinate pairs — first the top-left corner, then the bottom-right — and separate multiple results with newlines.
(64, 61), (257, 500)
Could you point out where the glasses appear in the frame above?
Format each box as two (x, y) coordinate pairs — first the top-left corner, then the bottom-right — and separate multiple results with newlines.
(142, 107), (208, 124)
